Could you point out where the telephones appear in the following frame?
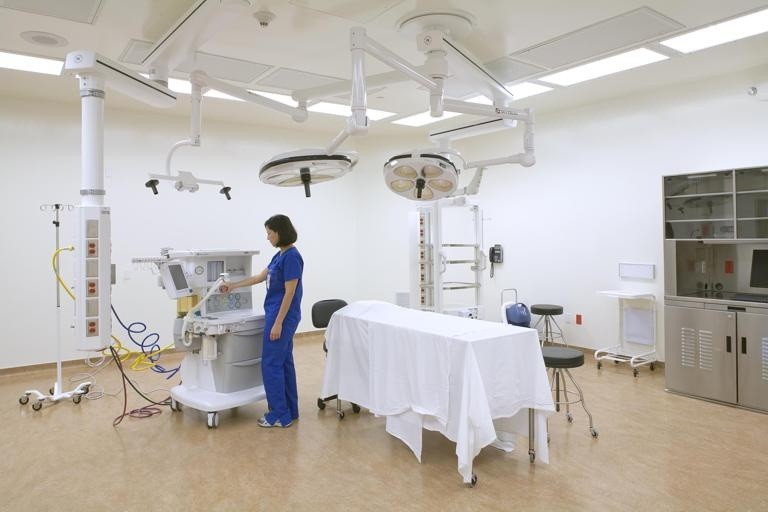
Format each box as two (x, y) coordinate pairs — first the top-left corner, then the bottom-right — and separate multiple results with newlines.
(489, 245), (503, 263)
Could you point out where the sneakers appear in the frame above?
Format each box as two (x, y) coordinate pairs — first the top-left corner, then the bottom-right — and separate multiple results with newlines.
(256, 411), (291, 427)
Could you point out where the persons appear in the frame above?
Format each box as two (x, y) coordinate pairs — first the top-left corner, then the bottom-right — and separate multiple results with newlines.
(218, 215), (304, 426)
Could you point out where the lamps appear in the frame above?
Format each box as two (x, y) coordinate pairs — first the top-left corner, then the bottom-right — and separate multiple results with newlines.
(257, 8), (536, 202)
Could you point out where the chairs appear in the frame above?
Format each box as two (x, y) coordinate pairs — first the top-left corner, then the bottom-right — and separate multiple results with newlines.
(311, 299), (361, 420)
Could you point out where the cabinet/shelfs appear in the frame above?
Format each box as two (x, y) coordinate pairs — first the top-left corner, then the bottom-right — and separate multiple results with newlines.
(663, 164), (768, 416)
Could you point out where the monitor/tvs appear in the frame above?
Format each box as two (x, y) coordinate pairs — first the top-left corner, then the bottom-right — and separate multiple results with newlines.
(749, 250), (768, 288)
(160, 262), (193, 299)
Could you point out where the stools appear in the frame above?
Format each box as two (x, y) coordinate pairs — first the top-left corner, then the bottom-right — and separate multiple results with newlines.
(529, 304), (598, 443)
